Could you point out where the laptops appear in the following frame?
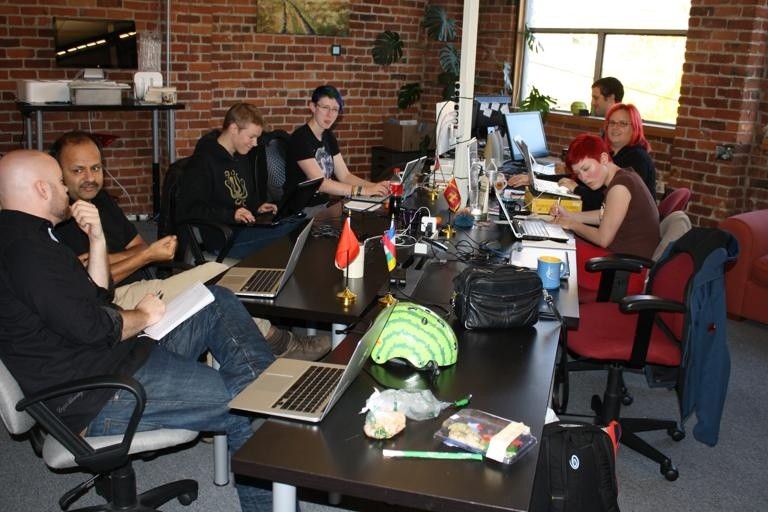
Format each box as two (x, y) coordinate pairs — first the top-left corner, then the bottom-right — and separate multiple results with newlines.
(352, 156), (428, 204)
(514, 137), (555, 178)
(491, 185), (569, 243)
(227, 302), (397, 423)
(251, 176), (324, 227)
(215, 218), (315, 299)
(521, 139), (581, 200)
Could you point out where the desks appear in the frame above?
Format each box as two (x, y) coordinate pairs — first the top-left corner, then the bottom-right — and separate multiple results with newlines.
(227, 296), (565, 512)
(370, 145), (436, 182)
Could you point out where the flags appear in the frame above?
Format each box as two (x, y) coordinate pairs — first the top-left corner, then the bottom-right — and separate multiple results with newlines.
(335, 215), (360, 270)
(380, 213), (396, 273)
(443, 178), (461, 213)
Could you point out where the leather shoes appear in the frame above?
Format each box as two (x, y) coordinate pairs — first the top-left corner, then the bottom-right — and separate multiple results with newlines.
(274, 332), (333, 361)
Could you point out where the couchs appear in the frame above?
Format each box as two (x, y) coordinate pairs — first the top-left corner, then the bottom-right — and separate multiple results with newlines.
(719, 207), (767, 327)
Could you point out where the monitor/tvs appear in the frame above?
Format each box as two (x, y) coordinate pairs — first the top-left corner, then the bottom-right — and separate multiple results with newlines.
(436, 100), (455, 158)
(504, 111), (550, 161)
(472, 95), (512, 148)
(53, 14), (138, 84)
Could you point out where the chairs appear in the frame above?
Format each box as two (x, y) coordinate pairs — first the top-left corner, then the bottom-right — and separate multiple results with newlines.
(577, 187), (693, 300)
(552, 226), (739, 483)
(1, 357), (204, 512)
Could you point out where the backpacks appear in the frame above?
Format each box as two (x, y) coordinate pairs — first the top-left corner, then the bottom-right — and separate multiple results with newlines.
(529, 419), (621, 511)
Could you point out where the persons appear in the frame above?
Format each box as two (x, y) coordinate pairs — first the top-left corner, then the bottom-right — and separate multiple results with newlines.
(50, 131), (331, 444)
(554, 79), (630, 175)
(509, 103), (656, 211)
(550, 133), (659, 296)
(177, 103), (292, 260)
(0, 148), (297, 512)
(284, 86), (392, 217)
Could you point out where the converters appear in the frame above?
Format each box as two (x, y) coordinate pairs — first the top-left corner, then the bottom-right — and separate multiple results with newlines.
(390, 267), (406, 284)
(421, 216), (437, 234)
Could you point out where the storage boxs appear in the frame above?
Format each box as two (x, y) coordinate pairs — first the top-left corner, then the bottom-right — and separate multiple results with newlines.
(214, 156), (582, 334)
(380, 118), (436, 152)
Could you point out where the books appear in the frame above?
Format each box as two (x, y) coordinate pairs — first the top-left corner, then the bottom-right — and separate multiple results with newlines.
(137, 280), (214, 343)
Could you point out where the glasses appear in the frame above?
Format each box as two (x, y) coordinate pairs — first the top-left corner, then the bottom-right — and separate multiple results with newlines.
(608, 118), (633, 127)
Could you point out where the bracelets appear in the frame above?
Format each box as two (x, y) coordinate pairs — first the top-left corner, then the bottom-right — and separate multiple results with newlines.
(351, 184), (366, 197)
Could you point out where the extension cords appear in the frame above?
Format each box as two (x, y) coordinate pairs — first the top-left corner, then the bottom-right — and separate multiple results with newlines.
(453, 81), (461, 138)
(414, 230), (438, 255)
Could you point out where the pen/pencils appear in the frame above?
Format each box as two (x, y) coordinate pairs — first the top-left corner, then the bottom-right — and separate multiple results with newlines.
(555, 196), (561, 219)
(156, 290), (161, 296)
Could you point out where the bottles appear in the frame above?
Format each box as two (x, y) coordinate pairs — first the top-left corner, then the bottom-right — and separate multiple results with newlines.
(388, 167), (404, 214)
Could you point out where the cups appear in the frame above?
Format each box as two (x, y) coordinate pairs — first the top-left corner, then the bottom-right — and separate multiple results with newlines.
(162, 87), (178, 105)
(538, 256), (566, 289)
(495, 180), (508, 194)
(499, 197), (522, 221)
(487, 130), (504, 168)
(341, 242), (365, 279)
(487, 170), (496, 193)
(561, 148), (568, 162)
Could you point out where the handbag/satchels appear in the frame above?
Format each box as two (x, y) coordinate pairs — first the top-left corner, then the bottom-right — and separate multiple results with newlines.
(450, 261), (544, 330)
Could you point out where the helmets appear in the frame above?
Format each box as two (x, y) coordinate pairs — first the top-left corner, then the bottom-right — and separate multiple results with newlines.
(367, 303), (459, 369)
